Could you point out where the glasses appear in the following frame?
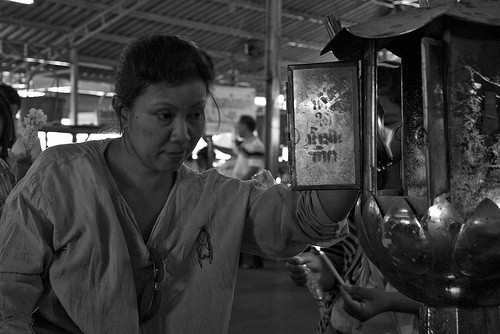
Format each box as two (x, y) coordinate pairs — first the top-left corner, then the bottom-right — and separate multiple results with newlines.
(137, 243), (165, 325)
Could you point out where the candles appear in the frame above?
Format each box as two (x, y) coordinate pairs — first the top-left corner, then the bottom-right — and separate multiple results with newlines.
(315, 246), (345, 287)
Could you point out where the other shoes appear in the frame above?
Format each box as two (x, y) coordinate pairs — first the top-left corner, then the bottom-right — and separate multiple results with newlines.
(248, 264), (264, 269)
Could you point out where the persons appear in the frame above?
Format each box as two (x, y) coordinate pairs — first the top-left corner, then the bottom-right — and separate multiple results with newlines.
(1, 33), (362, 334)
(0, 83), (422, 334)
